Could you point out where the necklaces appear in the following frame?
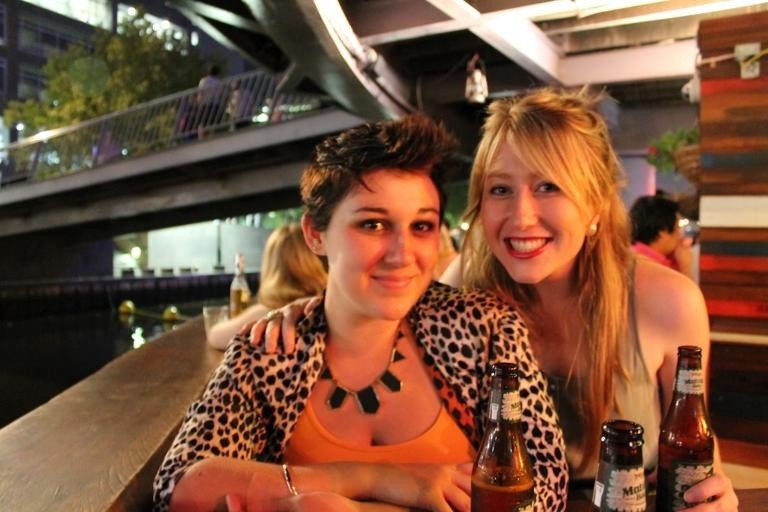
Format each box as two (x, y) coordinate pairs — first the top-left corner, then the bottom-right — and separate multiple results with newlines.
(317, 319), (407, 416)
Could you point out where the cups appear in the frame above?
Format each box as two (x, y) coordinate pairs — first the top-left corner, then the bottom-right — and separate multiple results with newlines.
(203, 306), (228, 336)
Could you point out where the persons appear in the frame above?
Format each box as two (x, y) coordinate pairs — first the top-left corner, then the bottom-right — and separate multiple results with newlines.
(194, 66), (225, 139)
(207, 223), (332, 351)
(223, 77), (257, 133)
(153, 111), (569, 511)
(622, 194), (694, 284)
(460, 52), (490, 156)
(236, 86), (742, 510)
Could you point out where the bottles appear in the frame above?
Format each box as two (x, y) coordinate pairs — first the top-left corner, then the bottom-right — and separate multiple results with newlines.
(655, 345), (714, 512)
(589, 419), (649, 512)
(470, 362), (536, 512)
(229, 253), (251, 320)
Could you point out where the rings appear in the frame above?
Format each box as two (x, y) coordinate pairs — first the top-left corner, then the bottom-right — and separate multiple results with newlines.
(266, 310), (283, 322)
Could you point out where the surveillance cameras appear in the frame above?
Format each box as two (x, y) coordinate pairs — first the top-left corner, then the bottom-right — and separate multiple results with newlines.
(681, 78), (700, 104)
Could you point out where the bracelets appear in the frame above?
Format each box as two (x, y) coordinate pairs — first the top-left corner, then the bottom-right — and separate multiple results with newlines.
(280, 463), (298, 497)
